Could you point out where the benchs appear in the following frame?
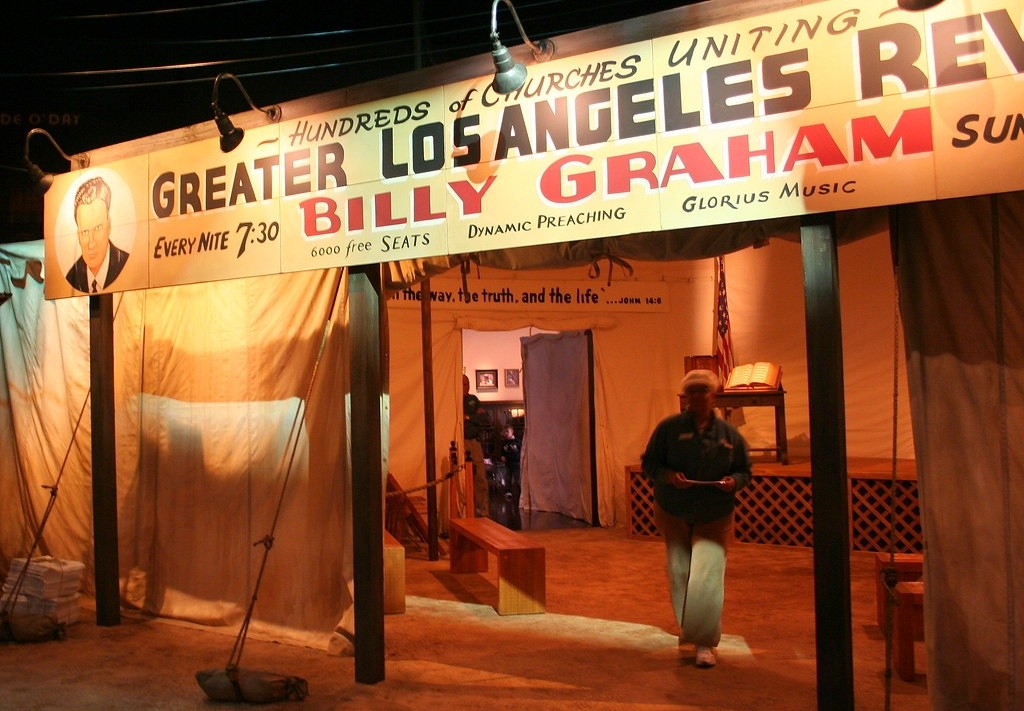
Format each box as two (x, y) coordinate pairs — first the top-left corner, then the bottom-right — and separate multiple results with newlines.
(891, 580), (925, 682)
(448, 515), (547, 616)
(874, 550), (923, 636)
(382, 528), (407, 615)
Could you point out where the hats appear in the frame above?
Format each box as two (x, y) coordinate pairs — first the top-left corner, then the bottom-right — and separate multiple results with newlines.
(682, 369), (720, 394)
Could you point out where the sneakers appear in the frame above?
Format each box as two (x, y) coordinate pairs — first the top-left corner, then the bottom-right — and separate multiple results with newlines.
(696, 644), (716, 666)
(678, 640), (697, 659)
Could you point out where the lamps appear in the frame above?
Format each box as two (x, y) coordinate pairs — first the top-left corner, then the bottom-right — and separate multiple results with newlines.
(210, 73), (281, 153)
(22, 127), (90, 195)
(490, 0), (555, 95)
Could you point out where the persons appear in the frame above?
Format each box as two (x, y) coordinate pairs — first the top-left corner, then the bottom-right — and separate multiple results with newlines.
(463, 374), (493, 517)
(498, 425), (521, 497)
(641, 369), (752, 666)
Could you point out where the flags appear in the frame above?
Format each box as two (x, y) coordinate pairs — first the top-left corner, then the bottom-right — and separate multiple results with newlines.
(718, 251), (747, 428)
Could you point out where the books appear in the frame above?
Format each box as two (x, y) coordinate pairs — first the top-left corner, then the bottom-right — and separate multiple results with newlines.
(1, 555), (86, 626)
(728, 361), (780, 388)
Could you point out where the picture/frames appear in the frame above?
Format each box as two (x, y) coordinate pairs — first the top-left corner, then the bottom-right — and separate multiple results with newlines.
(476, 369), (498, 390)
(504, 369), (519, 388)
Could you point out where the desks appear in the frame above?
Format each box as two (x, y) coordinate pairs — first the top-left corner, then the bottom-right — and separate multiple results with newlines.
(677, 383), (789, 465)
